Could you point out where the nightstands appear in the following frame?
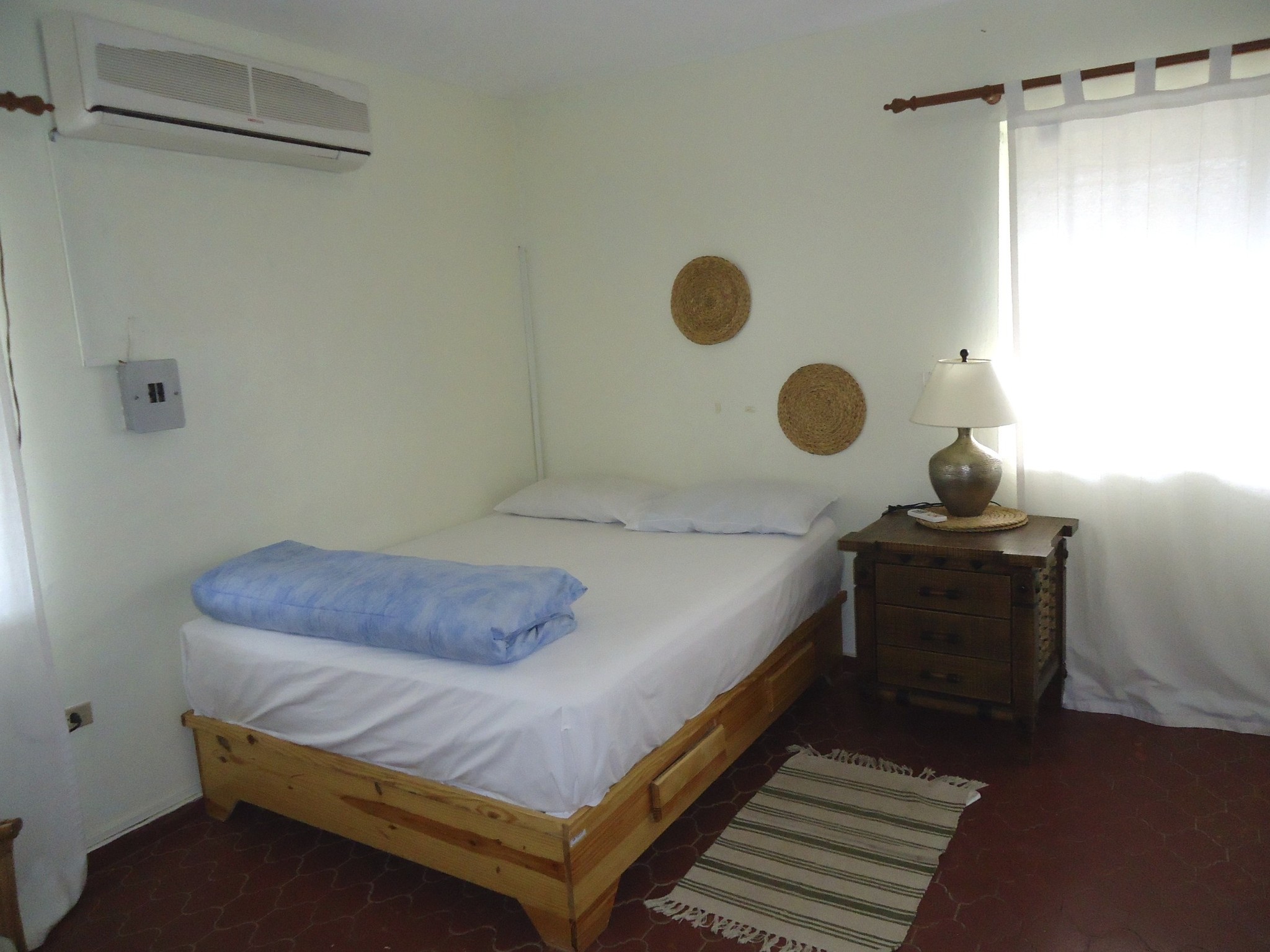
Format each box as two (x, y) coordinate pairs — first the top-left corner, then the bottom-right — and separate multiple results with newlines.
(837, 502), (1080, 737)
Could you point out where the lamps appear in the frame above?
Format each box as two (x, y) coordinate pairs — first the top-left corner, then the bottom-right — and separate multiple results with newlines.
(910, 348), (1009, 516)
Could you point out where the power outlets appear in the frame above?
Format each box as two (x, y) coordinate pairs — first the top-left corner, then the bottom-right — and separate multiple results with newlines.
(66, 700), (93, 731)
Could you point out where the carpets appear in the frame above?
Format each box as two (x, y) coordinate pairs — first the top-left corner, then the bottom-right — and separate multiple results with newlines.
(640, 741), (988, 951)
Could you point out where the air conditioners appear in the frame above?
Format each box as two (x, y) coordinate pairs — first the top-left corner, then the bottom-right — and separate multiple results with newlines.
(38, 11), (373, 170)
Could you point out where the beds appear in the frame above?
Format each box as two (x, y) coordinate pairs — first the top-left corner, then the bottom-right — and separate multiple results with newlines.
(181, 516), (851, 951)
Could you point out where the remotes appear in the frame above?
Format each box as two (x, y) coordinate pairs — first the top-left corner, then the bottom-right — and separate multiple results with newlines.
(907, 509), (947, 523)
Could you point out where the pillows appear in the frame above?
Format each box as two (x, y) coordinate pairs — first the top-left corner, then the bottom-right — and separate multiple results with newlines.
(626, 486), (840, 535)
(494, 477), (666, 523)
